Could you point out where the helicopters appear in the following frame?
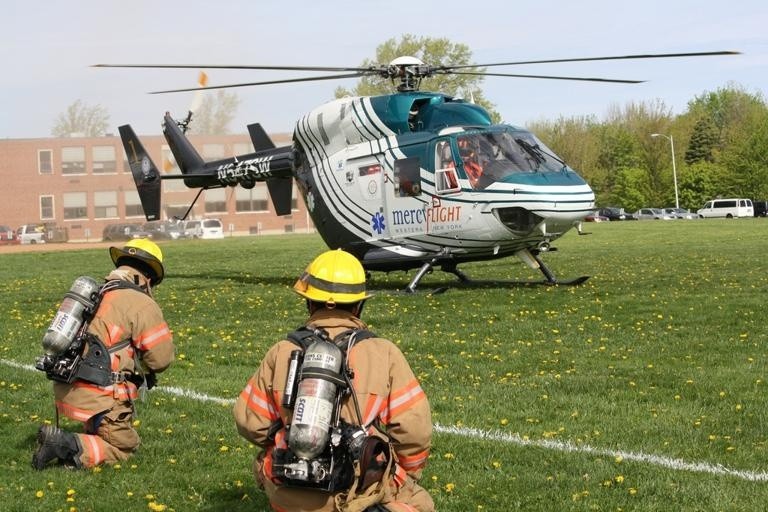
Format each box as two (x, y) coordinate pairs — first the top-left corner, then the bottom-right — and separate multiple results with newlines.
(86, 46), (744, 297)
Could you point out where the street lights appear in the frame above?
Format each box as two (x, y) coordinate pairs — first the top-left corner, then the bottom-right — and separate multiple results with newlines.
(649, 132), (680, 209)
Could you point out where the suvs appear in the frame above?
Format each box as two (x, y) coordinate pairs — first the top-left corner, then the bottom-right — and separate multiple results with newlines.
(139, 220), (192, 241)
(99, 220), (155, 242)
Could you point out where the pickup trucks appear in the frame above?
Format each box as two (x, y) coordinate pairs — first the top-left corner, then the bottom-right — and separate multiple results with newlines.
(11, 223), (70, 245)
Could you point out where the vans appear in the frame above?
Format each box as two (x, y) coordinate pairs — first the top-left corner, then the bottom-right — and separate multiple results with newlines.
(179, 217), (225, 239)
(696, 198), (754, 218)
(751, 199), (768, 216)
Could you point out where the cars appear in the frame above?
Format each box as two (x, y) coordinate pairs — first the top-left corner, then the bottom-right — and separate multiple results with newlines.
(582, 204), (701, 222)
(0, 223), (22, 247)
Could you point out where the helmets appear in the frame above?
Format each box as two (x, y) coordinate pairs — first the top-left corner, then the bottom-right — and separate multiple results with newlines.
(294, 249), (373, 306)
(110, 238), (163, 284)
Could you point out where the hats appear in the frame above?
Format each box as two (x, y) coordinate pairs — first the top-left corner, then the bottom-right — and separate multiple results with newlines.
(457, 139), (474, 152)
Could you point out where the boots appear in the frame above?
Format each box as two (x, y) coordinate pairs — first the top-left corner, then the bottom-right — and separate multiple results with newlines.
(34, 424), (75, 470)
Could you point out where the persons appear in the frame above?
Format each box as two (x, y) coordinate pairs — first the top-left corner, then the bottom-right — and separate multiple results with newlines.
(31, 236), (176, 470)
(451, 138), (486, 190)
(233, 247), (441, 512)
